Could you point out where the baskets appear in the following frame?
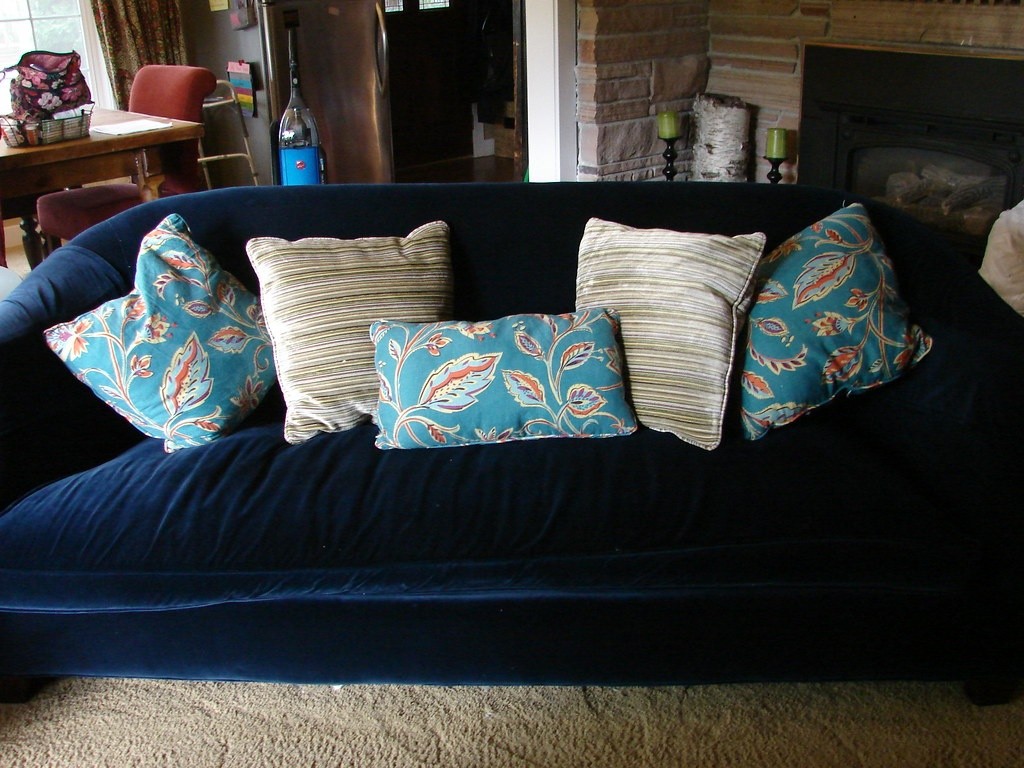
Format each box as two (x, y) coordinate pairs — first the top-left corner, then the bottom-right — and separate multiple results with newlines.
(0, 101), (95, 148)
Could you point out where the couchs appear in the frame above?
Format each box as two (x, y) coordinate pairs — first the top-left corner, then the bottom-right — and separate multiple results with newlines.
(0, 182), (1024, 707)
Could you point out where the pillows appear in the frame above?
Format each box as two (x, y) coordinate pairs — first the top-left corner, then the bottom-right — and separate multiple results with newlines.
(728, 203), (933, 441)
(245, 220), (451, 443)
(45, 213), (277, 453)
(369, 307), (637, 450)
(575, 216), (767, 452)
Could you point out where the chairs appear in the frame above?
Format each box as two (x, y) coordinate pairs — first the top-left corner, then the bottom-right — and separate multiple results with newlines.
(0, 64), (216, 270)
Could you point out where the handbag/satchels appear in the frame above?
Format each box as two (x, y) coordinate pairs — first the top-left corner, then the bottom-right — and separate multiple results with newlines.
(0, 49), (91, 123)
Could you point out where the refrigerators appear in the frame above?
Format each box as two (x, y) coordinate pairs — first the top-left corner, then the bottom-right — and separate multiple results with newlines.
(185, 0), (398, 185)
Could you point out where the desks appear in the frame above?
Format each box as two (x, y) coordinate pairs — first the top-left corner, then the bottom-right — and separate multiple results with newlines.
(0, 104), (205, 271)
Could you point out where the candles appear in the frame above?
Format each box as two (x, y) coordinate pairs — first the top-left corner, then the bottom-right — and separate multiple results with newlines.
(658, 110), (680, 139)
(766, 128), (787, 158)
